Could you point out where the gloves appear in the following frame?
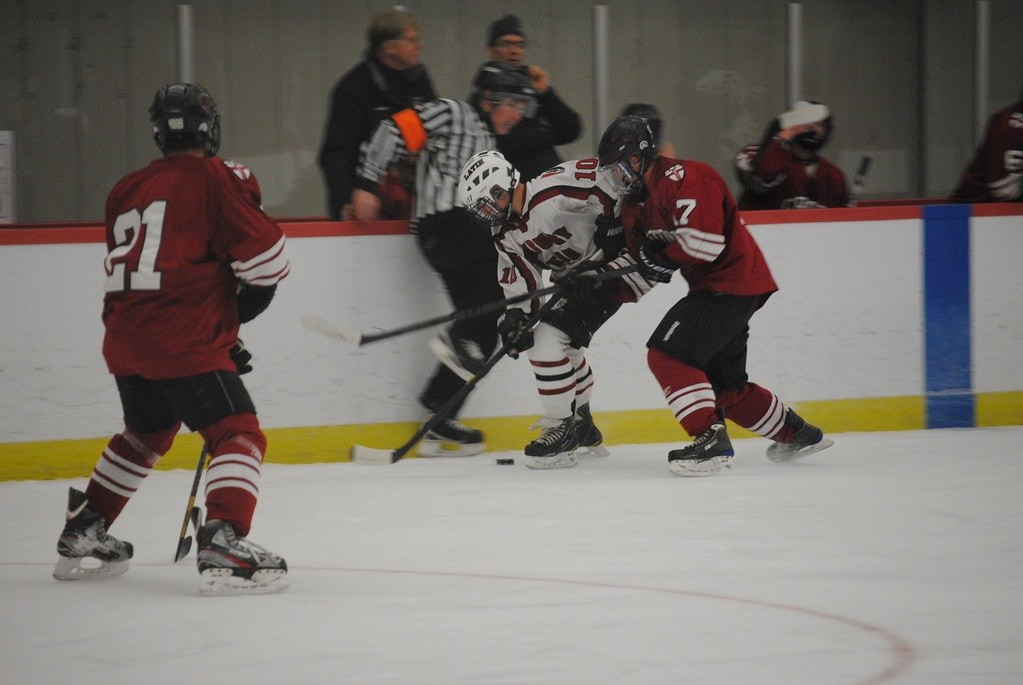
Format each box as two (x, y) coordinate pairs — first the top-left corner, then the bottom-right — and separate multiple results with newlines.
(561, 264), (612, 305)
(593, 213), (624, 260)
(498, 308), (535, 352)
(228, 337), (253, 375)
(639, 241), (681, 283)
(237, 280), (278, 324)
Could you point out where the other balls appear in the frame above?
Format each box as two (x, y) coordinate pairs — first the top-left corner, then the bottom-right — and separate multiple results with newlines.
(497, 458), (514, 466)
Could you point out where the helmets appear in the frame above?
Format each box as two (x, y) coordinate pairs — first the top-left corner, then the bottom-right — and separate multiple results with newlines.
(781, 101), (833, 152)
(469, 60), (541, 121)
(457, 148), (520, 221)
(597, 114), (655, 217)
(149, 84), (221, 160)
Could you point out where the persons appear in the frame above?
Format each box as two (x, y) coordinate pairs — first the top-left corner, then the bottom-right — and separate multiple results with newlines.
(741, 101), (850, 211)
(51, 81), (289, 594)
(465, 14), (582, 184)
(951, 102), (1023, 202)
(318, 7), (440, 221)
(353, 61), (837, 480)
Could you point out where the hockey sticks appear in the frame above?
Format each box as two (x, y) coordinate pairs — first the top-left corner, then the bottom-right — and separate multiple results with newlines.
(173, 281), (247, 563)
(301, 263), (644, 348)
(846, 154), (873, 208)
(349, 291), (563, 466)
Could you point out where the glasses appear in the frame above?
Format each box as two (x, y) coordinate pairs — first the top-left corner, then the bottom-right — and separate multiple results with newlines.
(494, 40), (526, 49)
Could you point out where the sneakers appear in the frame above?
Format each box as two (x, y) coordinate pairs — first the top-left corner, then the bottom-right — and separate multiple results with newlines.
(416, 408), (484, 458)
(428, 324), (486, 382)
(576, 400), (609, 459)
(766, 406), (835, 465)
(191, 506), (289, 596)
(524, 398), (578, 468)
(667, 407), (734, 475)
(51, 486), (135, 581)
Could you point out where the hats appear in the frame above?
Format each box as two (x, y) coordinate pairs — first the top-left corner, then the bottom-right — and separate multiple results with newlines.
(486, 14), (526, 46)
